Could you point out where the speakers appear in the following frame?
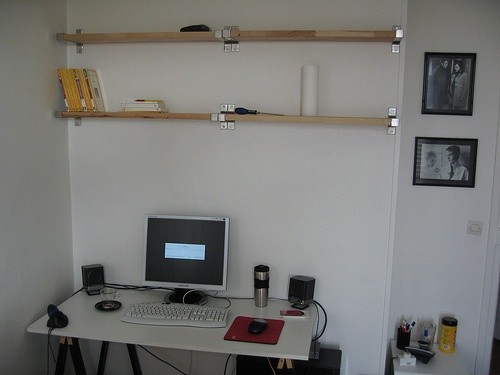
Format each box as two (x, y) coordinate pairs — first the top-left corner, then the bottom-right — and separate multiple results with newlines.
(288, 275), (315, 305)
(82, 264), (104, 293)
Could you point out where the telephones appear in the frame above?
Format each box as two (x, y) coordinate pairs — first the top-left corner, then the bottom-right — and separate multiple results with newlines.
(405, 340), (437, 364)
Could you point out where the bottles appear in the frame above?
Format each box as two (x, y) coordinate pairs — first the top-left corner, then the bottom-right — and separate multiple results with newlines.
(438, 317), (458, 353)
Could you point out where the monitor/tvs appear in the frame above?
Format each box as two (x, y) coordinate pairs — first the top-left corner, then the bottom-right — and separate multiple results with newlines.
(144, 214), (228, 306)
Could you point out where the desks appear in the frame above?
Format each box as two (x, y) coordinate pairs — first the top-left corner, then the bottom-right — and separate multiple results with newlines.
(390, 338), (475, 375)
(27, 287), (316, 375)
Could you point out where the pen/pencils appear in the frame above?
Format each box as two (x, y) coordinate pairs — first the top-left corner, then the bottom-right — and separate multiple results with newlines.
(400, 319), (416, 332)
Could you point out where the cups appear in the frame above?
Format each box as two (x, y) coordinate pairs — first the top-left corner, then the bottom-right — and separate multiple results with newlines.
(254, 264), (270, 308)
(100, 288), (116, 308)
(417, 320), (437, 350)
(397, 328), (411, 350)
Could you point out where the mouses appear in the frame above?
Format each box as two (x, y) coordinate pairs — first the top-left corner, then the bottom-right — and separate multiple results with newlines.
(248, 318), (269, 333)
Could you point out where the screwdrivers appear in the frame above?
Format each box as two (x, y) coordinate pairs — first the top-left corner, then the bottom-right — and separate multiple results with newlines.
(234, 107), (285, 116)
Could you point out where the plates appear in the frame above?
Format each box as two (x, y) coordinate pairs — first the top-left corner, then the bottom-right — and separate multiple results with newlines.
(95, 300), (122, 311)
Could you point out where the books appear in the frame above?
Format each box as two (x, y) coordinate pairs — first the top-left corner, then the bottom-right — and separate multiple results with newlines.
(56, 67), (168, 112)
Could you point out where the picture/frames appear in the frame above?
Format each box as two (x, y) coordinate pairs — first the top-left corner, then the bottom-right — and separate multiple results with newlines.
(413, 137), (479, 188)
(421, 52), (477, 117)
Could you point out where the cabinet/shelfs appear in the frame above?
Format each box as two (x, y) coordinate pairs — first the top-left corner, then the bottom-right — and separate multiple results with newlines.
(53, 25), (404, 135)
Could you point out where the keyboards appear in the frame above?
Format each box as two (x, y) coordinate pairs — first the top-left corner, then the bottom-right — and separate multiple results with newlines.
(123, 302), (229, 329)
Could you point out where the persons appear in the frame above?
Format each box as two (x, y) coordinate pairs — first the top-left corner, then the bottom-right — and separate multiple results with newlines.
(432, 56), (468, 110)
(437, 145), (468, 179)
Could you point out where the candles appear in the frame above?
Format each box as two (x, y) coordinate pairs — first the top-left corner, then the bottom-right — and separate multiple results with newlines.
(300, 63), (318, 116)
